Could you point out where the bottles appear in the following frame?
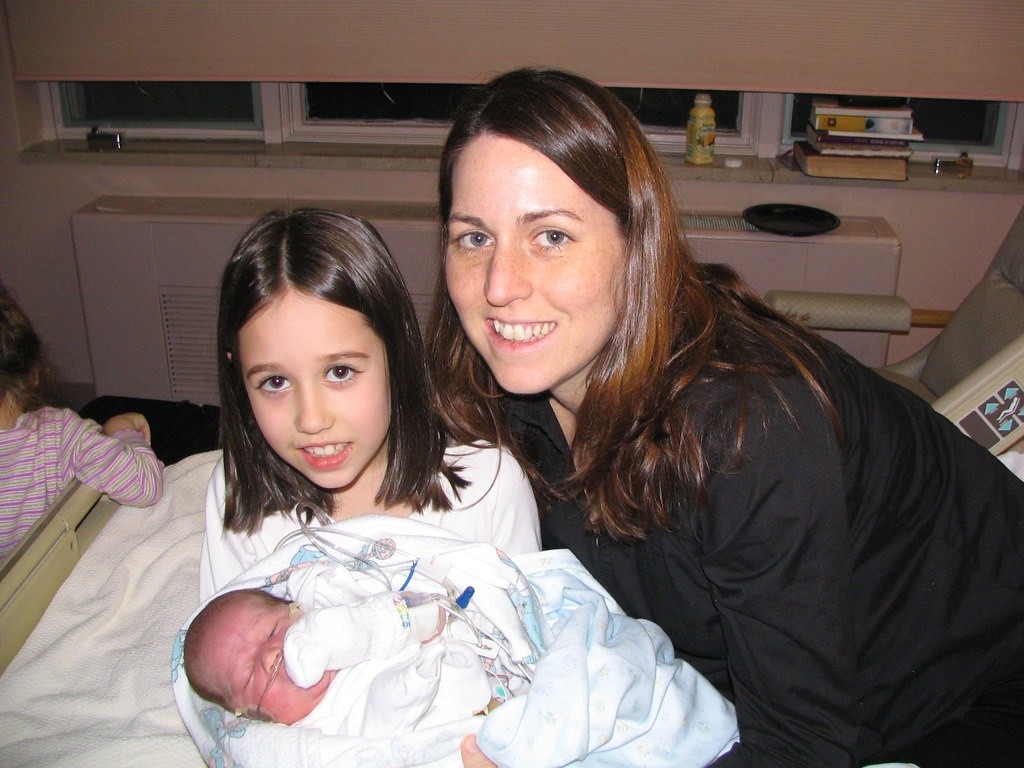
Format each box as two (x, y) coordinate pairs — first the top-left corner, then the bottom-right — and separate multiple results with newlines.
(684, 94), (716, 166)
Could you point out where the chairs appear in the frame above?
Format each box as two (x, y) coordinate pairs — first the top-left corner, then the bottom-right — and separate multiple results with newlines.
(759, 207), (1023, 457)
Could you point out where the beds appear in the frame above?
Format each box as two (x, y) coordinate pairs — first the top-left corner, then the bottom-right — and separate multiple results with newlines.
(0, 415), (1023, 768)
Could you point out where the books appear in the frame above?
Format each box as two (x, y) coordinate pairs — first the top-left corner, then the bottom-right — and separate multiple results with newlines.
(793, 98), (926, 181)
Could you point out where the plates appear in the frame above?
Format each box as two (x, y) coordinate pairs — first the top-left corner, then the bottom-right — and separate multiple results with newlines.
(742, 203), (841, 237)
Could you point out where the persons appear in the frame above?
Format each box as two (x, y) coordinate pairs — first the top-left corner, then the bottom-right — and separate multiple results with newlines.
(423, 68), (1024, 768)
(172, 514), (740, 768)
(197, 205), (543, 605)
(0, 281), (165, 567)
